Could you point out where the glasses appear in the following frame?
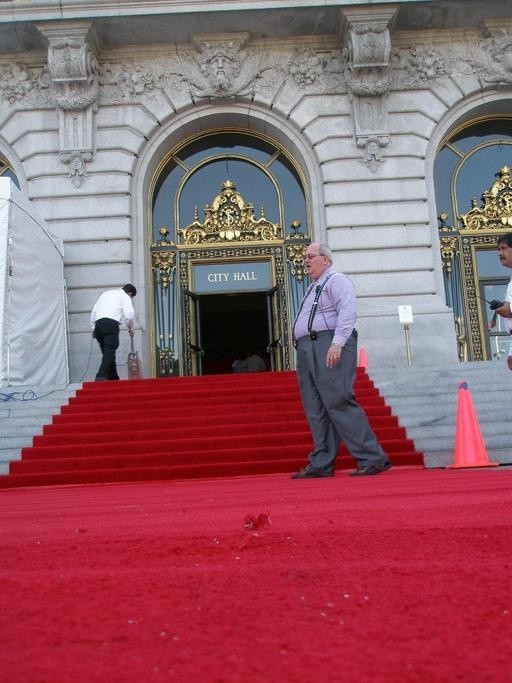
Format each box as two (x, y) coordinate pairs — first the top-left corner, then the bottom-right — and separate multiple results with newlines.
(303, 253), (325, 261)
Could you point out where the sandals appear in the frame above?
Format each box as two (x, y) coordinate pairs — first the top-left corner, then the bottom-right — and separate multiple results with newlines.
(350, 459), (392, 475)
(291, 469), (334, 479)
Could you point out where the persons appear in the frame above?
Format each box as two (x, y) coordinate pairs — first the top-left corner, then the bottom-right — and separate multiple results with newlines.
(495, 232), (512, 371)
(290, 241), (394, 480)
(89, 283), (137, 381)
(200, 46), (241, 90)
(231, 345), (271, 373)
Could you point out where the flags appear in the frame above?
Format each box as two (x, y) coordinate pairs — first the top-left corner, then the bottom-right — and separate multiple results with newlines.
(488, 312), (497, 332)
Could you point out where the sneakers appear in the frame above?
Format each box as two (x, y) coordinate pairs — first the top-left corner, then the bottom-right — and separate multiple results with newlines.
(95, 377), (108, 381)
